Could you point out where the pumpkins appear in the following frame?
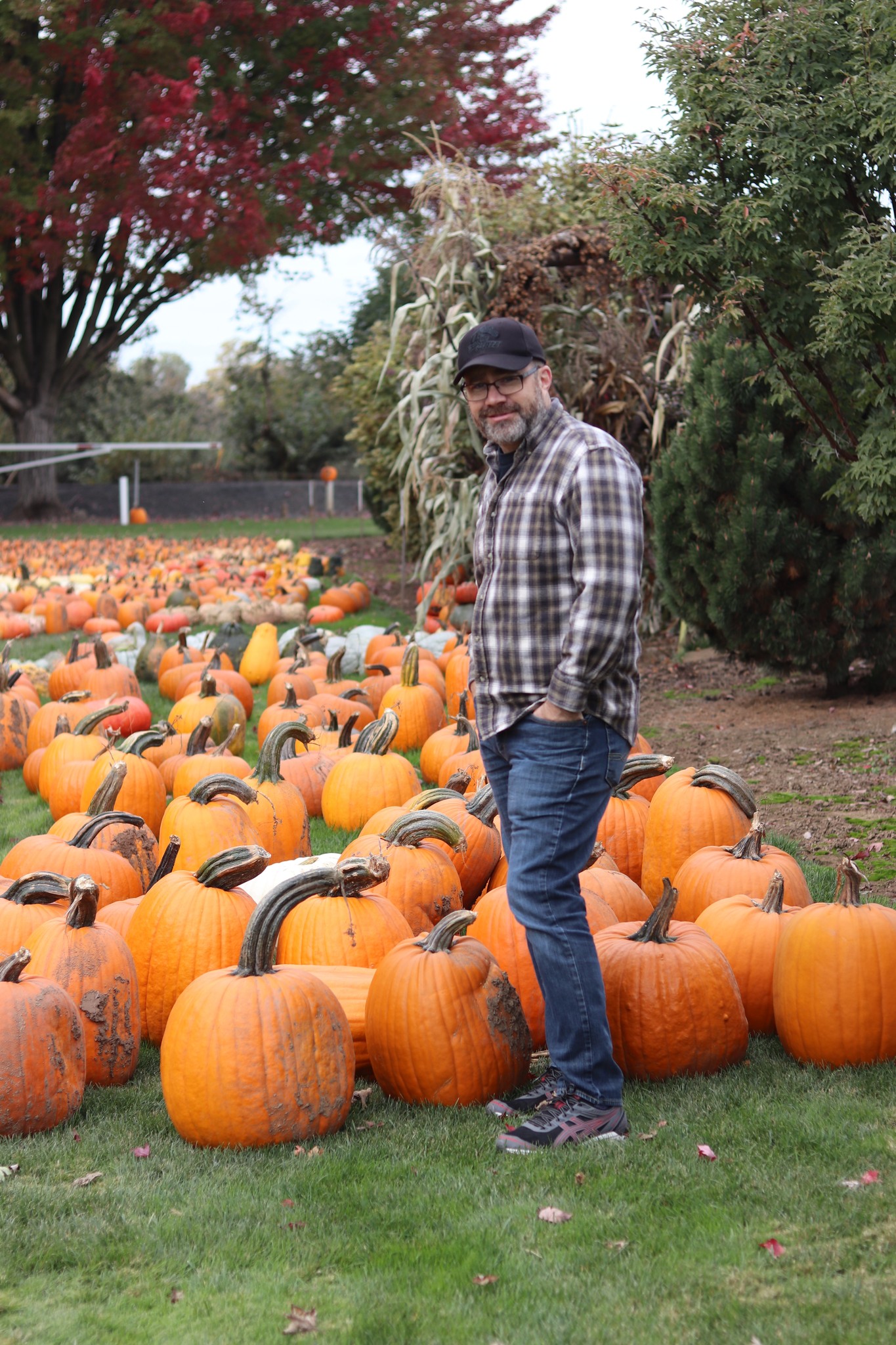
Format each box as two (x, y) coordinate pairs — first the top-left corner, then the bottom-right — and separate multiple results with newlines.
(0, 463), (894, 1148)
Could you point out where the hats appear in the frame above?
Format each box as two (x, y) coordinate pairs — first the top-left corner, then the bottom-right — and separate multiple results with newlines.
(451, 316), (548, 386)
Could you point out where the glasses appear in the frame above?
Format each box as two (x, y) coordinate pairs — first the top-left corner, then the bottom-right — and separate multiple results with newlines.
(458, 366), (548, 403)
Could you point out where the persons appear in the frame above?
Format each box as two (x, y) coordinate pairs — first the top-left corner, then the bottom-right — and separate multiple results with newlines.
(449, 320), (643, 1154)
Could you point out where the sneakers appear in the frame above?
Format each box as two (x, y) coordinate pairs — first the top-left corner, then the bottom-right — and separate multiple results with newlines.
(496, 1092), (633, 1158)
(486, 1067), (583, 1119)
